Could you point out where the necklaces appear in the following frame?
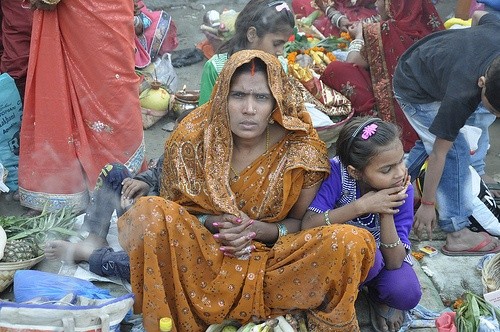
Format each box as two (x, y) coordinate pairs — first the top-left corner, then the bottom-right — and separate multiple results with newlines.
(355, 213), (375, 226)
(228, 124), (270, 178)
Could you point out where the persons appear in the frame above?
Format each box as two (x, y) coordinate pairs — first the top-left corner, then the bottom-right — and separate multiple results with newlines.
(44, 153), (166, 285)
(318, 0), (447, 155)
(131, 0), (378, 72)
(198, 0), (296, 111)
(389, 8), (499, 257)
(300, 113), (423, 331)
(17, 0), (150, 215)
(114, 50), (377, 331)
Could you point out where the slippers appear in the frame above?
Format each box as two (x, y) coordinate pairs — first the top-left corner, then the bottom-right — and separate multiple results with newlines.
(440, 231), (500, 256)
(368, 293), (404, 332)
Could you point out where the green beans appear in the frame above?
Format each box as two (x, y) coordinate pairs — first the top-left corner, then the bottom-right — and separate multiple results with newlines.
(10, 199), (82, 240)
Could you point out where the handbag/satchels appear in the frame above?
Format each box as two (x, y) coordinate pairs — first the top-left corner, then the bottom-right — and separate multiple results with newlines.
(139, 54), (177, 97)
(1, 294), (134, 331)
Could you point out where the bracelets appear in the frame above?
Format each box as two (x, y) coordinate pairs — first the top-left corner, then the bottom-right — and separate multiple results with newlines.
(42, 0), (61, 4)
(324, 208), (331, 226)
(380, 238), (401, 248)
(346, 38), (365, 53)
(195, 213), (210, 225)
(420, 198), (436, 206)
(265, 222), (289, 248)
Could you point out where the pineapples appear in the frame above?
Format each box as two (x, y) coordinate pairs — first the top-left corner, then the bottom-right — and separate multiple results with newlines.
(3, 241), (35, 262)
